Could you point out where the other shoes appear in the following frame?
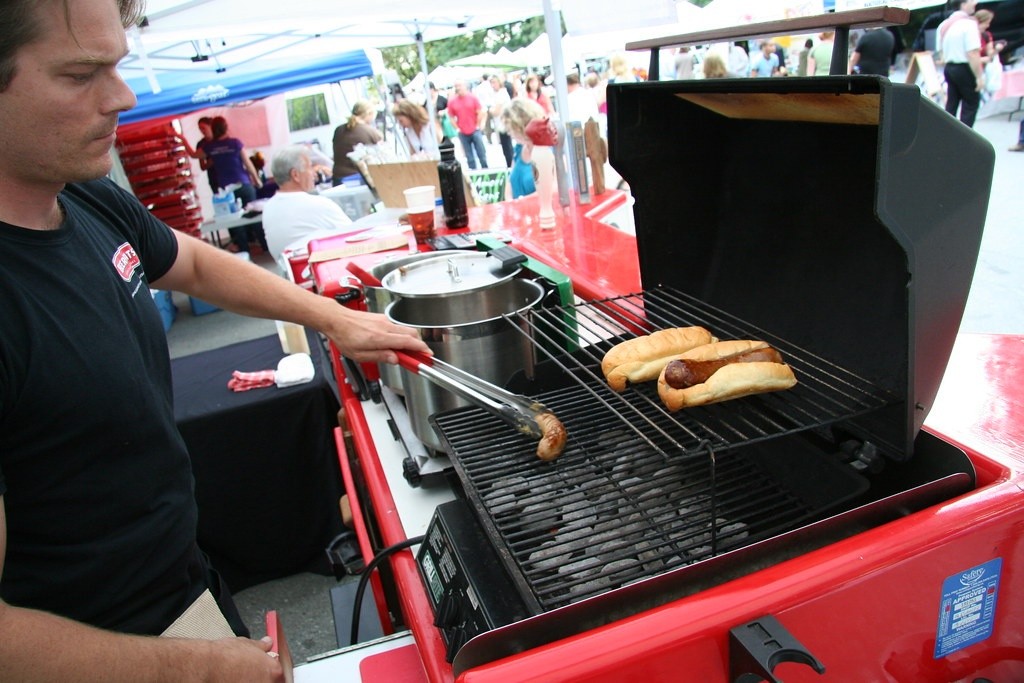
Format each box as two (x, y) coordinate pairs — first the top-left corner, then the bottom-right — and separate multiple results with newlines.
(251, 245), (263, 256)
(1009, 142), (1024, 152)
(227, 242), (237, 253)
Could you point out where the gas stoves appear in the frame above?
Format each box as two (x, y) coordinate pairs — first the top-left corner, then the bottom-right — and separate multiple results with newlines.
(368, 369), (455, 488)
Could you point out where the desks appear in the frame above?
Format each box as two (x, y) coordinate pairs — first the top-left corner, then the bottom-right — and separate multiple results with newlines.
(197, 209), (262, 248)
(170, 329), (340, 596)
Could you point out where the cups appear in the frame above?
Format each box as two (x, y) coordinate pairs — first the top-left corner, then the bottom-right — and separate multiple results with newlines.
(402, 185), (436, 231)
(406, 207), (434, 244)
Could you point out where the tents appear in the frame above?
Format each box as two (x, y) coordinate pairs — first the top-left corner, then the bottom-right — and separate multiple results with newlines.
(118, 0), (577, 192)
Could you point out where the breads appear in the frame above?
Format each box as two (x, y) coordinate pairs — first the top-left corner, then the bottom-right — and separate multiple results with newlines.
(602, 326), (718, 393)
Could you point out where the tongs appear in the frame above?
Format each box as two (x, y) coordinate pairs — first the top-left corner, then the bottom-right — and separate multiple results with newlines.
(392, 348), (555, 438)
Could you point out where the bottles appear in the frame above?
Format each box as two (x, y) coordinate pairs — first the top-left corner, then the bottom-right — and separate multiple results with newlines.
(436, 135), (469, 230)
(275, 319), (311, 357)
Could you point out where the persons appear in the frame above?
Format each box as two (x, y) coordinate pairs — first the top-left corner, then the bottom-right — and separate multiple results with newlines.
(260, 144), (360, 272)
(391, 100), (473, 193)
(333, 101), (383, 186)
(499, 99), (558, 204)
(0, 0), (434, 682)
(172, 117), (263, 208)
(416, 0), (1024, 168)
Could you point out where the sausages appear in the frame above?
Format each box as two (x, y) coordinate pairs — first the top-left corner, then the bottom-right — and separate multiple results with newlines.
(531, 412), (568, 461)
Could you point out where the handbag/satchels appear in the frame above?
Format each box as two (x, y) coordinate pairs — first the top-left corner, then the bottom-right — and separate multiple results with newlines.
(984, 54), (1003, 93)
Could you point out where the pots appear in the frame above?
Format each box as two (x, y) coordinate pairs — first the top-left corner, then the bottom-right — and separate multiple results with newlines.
(358, 248), (545, 456)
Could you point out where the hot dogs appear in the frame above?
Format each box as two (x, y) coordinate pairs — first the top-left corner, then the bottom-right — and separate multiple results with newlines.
(657, 341), (797, 411)
